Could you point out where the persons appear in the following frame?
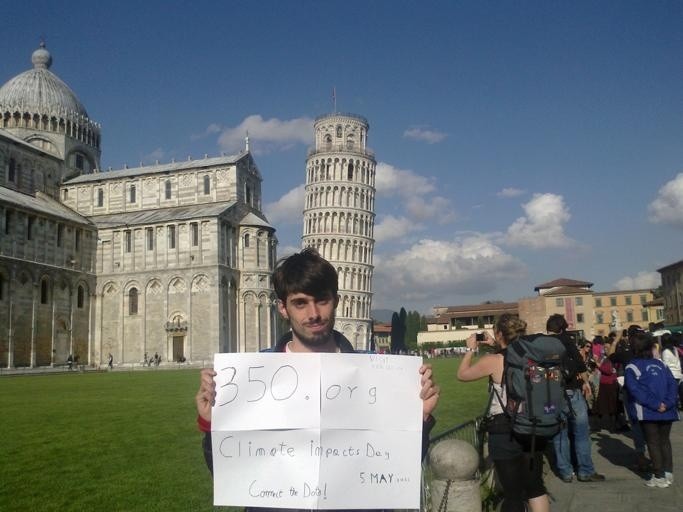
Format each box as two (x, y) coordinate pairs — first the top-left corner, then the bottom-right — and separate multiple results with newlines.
(371, 346), (466, 359)
(575, 321), (683, 467)
(456, 313), (550, 511)
(624, 334), (679, 489)
(153, 352), (159, 366)
(195, 245), (440, 511)
(143, 352), (149, 367)
(545, 314), (605, 482)
(67, 354), (73, 370)
(108, 353), (113, 369)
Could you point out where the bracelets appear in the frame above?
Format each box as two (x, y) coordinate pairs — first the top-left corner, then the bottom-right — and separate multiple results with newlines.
(466, 348), (475, 353)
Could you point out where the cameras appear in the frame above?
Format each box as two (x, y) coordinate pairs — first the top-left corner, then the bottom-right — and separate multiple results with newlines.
(476, 334), (485, 342)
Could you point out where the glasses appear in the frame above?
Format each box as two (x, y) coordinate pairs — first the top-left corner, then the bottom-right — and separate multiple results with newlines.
(586, 345), (592, 349)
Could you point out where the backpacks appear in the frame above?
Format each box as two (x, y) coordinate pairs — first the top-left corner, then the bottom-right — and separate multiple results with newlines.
(489, 333), (570, 438)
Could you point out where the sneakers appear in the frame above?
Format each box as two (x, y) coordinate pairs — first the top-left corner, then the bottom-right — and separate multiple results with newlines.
(632, 455), (653, 468)
(559, 472), (576, 483)
(642, 471), (674, 488)
(577, 472), (605, 482)
